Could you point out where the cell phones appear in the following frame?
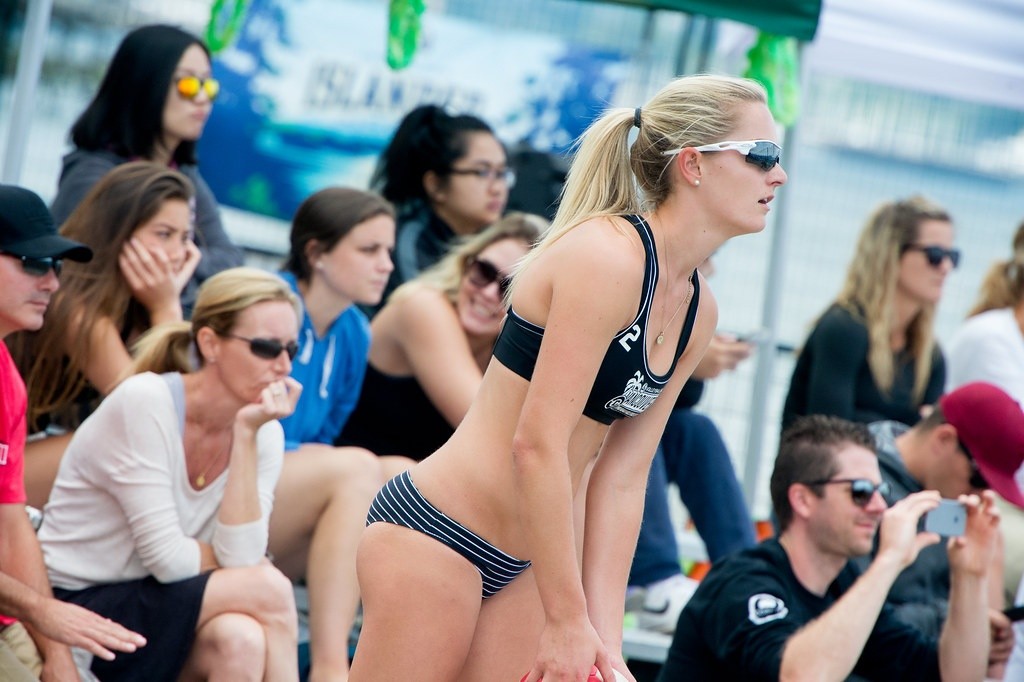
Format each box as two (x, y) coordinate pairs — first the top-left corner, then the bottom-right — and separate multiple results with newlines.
(923, 497), (969, 538)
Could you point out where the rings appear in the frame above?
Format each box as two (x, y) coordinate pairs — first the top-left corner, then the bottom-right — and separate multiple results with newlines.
(272, 390), (280, 396)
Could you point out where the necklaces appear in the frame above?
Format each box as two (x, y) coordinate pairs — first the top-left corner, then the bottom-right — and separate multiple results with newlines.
(196, 449), (223, 487)
(656, 209), (692, 344)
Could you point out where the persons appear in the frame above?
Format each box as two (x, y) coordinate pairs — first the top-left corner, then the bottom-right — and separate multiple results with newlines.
(0, 27), (1024, 682)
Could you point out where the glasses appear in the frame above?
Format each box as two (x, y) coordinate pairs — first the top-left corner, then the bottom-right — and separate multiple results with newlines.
(653, 141), (782, 172)
(898, 243), (960, 269)
(957, 439), (989, 489)
(169, 75), (220, 99)
(220, 332), (298, 361)
(448, 168), (516, 190)
(810, 477), (890, 509)
(22, 256), (63, 277)
(464, 257), (512, 302)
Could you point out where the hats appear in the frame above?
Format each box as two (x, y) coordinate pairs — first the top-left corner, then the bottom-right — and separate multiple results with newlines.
(941, 381), (1024, 511)
(0, 185), (93, 263)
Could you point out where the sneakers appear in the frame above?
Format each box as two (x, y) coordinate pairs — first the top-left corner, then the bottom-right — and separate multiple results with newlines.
(636, 572), (703, 633)
